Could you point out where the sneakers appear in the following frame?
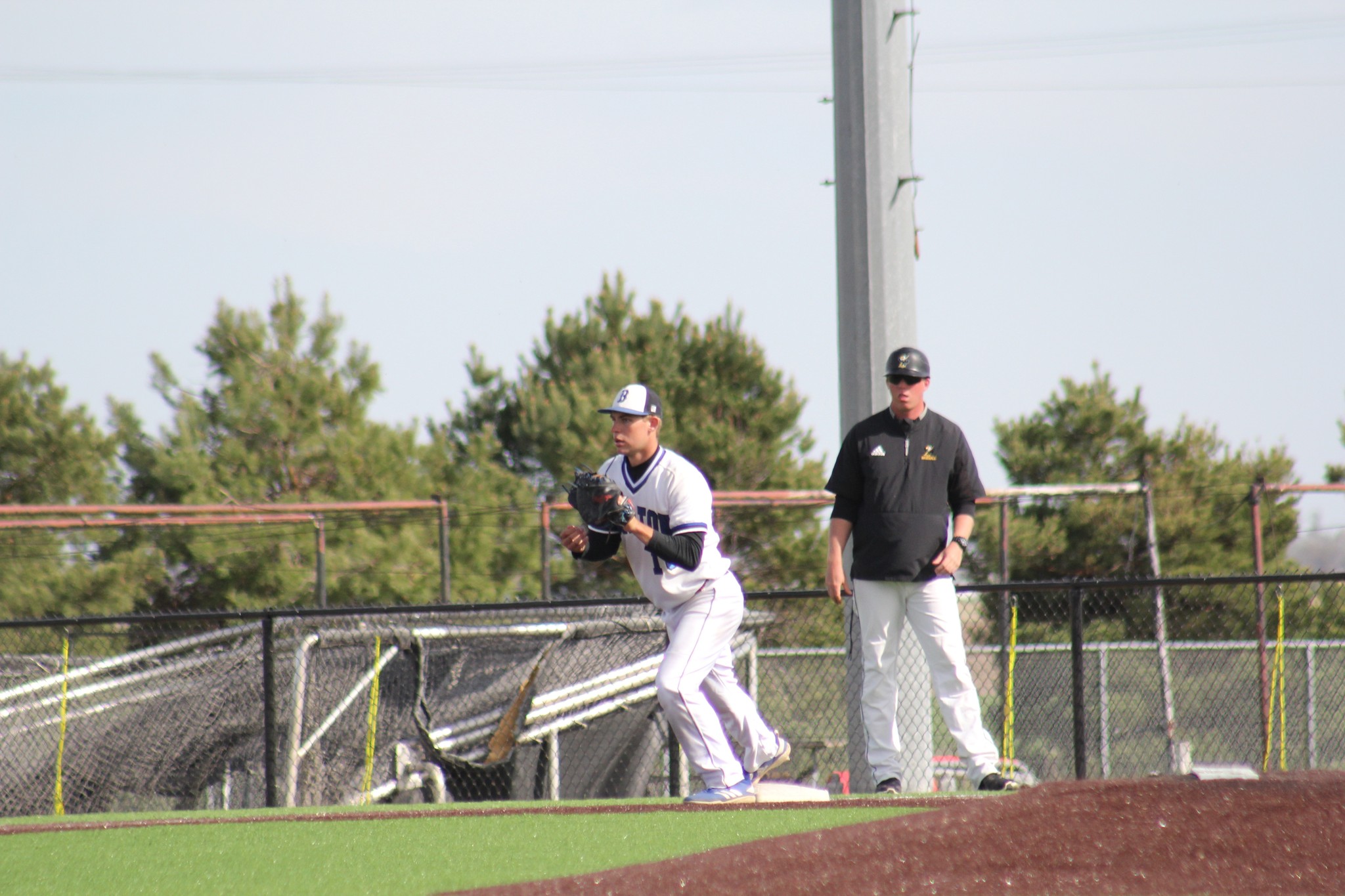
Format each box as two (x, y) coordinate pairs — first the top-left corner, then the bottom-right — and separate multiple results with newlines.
(683, 772), (756, 803)
(743, 730), (791, 785)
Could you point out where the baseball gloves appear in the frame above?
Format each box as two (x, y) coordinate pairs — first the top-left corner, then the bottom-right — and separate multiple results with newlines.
(561, 461), (638, 544)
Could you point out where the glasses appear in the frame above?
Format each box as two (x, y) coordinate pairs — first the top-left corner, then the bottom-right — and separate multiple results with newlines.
(887, 376), (925, 386)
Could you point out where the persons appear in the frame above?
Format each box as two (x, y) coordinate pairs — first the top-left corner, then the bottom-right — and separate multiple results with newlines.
(560, 384), (790, 804)
(823, 348), (1020, 792)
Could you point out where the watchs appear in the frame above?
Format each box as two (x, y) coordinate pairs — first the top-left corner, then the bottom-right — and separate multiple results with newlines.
(951, 536), (968, 552)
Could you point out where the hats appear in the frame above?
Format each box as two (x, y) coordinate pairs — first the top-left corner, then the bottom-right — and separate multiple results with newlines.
(883, 347), (930, 378)
(596, 384), (662, 416)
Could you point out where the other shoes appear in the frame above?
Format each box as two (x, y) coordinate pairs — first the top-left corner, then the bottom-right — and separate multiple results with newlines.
(978, 773), (1016, 791)
(874, 778), (901, 794)
